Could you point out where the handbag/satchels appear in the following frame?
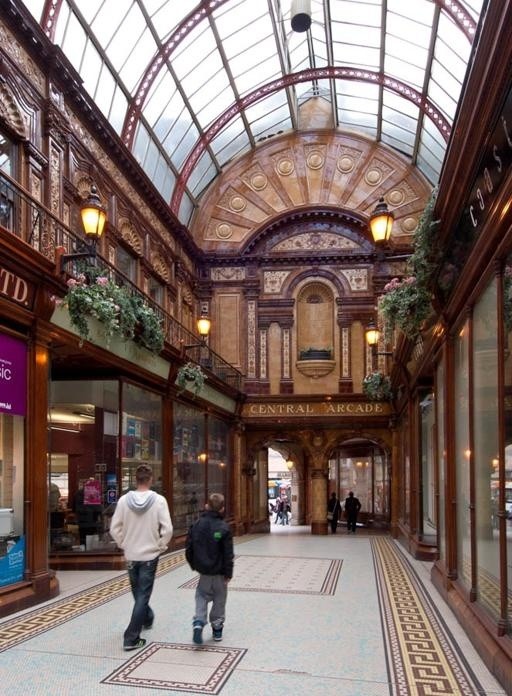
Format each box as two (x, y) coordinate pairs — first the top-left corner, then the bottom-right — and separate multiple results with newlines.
(327, 512), (334, 520)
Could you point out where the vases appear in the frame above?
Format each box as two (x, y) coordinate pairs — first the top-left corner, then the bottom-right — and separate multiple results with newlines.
(185, 372), (196, 381)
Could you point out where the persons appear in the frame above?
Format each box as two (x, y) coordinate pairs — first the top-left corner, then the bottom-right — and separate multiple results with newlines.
(107, 460), (174, 651)
(182, 492), (235, 644)
(326, 491), (343, 534)
(343, 491), (362, 533)
(71, 486), (96, 548)
(48, 480), (60, 551)
(272, 494), (292, 526)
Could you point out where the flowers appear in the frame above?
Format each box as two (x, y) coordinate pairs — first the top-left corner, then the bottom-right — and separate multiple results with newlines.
(176, 361), (208, 401)
(46, 266), (167, 359)
(363, 371), (392, 399)
(373, 278), (436, 341)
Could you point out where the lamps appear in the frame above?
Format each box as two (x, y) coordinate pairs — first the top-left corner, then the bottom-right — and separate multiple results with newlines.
(60, 185), (109, 273)
(370, 195), (412, 262)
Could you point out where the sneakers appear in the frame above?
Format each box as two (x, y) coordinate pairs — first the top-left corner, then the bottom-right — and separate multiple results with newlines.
(193, 622), (204, 644)
(123, 638), (146, 650)
(213, 627), (223, 641)
